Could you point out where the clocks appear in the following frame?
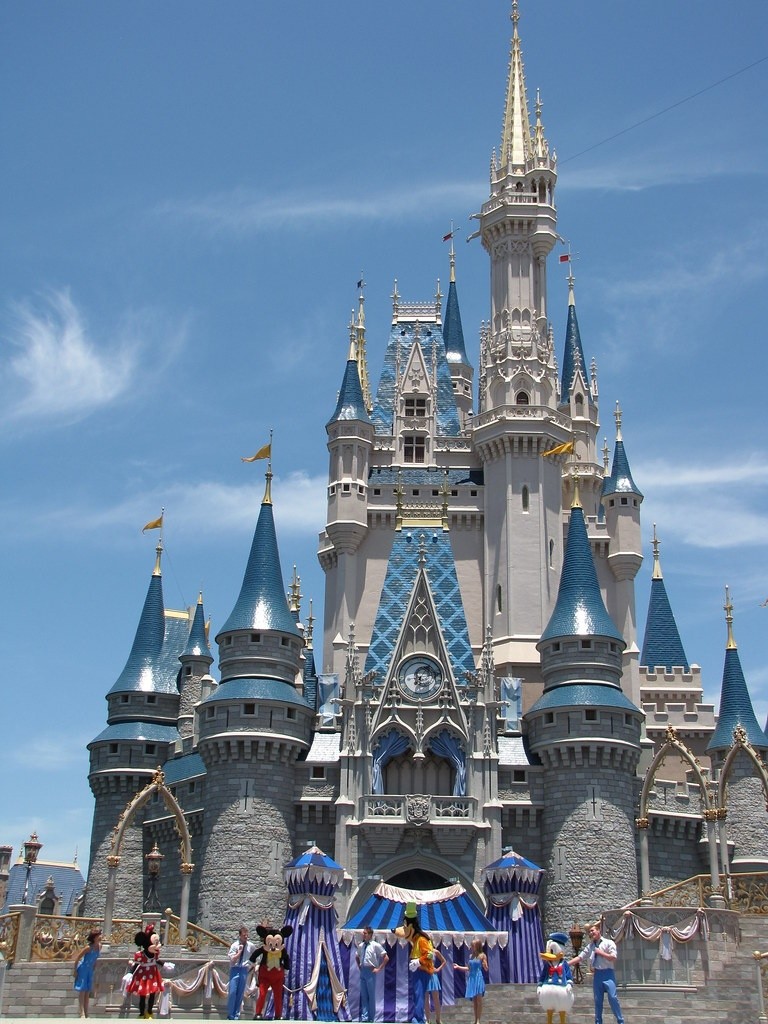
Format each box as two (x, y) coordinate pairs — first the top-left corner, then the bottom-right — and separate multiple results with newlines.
(395, 651), (446, 704)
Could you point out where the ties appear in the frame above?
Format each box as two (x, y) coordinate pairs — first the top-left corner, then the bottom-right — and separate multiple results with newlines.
(239, 943), (245, 966)
(362, 943), (368, 961)
(592, 940), (602, 966)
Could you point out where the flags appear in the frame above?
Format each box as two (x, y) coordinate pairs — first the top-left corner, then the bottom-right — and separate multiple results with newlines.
(142, 517), (162, 535)
(241, 444), (269, 462)
(539, 442), (573, 457)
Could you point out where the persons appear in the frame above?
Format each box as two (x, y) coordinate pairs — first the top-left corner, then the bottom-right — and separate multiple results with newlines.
(73, 930), (102, 1018)
(567, 925), (625, 1024)
(355, 927), (389, 1023)
(226, 927), (260, 1020)
(425, 931), (446, 1024)
(453, 939), (489, 1024)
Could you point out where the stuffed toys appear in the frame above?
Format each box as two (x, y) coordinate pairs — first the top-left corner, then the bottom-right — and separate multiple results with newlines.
(128, 925), (175, 1019)
(537, 933), (574, 1024)
(391, 902), (434, 1024)
(244, 925), (293, 1020)
